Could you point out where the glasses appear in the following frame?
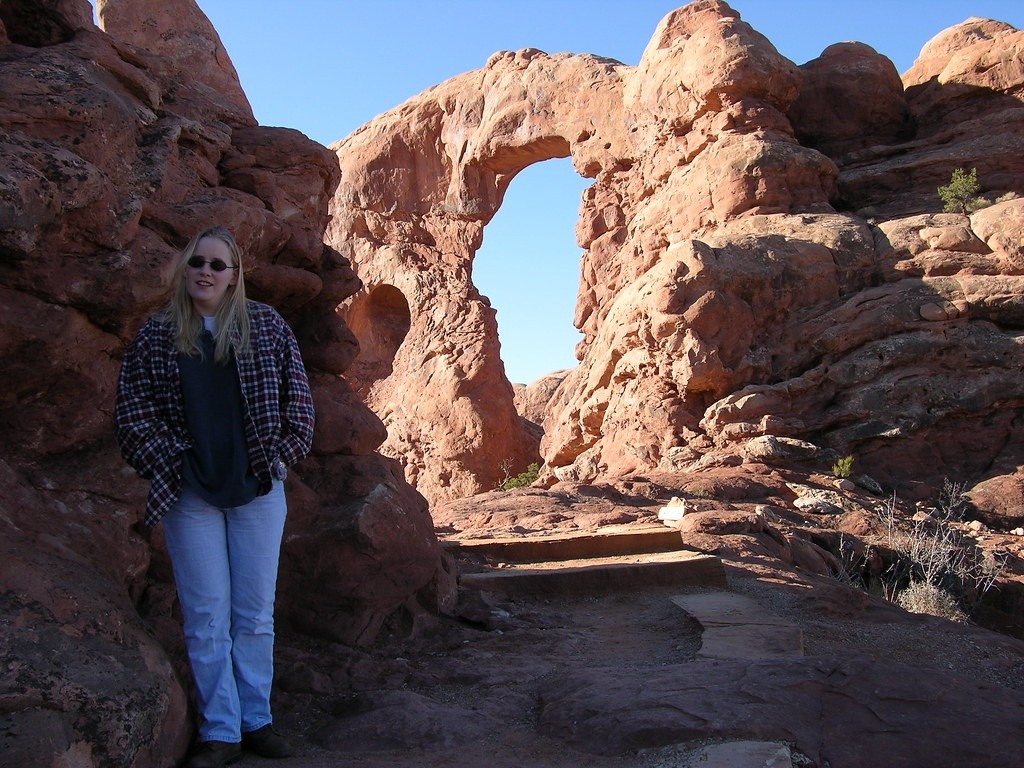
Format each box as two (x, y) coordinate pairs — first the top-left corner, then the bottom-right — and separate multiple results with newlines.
(188, 257), (237, 272)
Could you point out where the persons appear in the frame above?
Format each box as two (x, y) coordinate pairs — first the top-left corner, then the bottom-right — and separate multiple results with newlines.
(114, 226), (316, 768)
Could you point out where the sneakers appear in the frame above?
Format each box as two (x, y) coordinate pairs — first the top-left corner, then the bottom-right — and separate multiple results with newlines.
(188, 740), (243, 768)
(241, 723), (294, 758)
